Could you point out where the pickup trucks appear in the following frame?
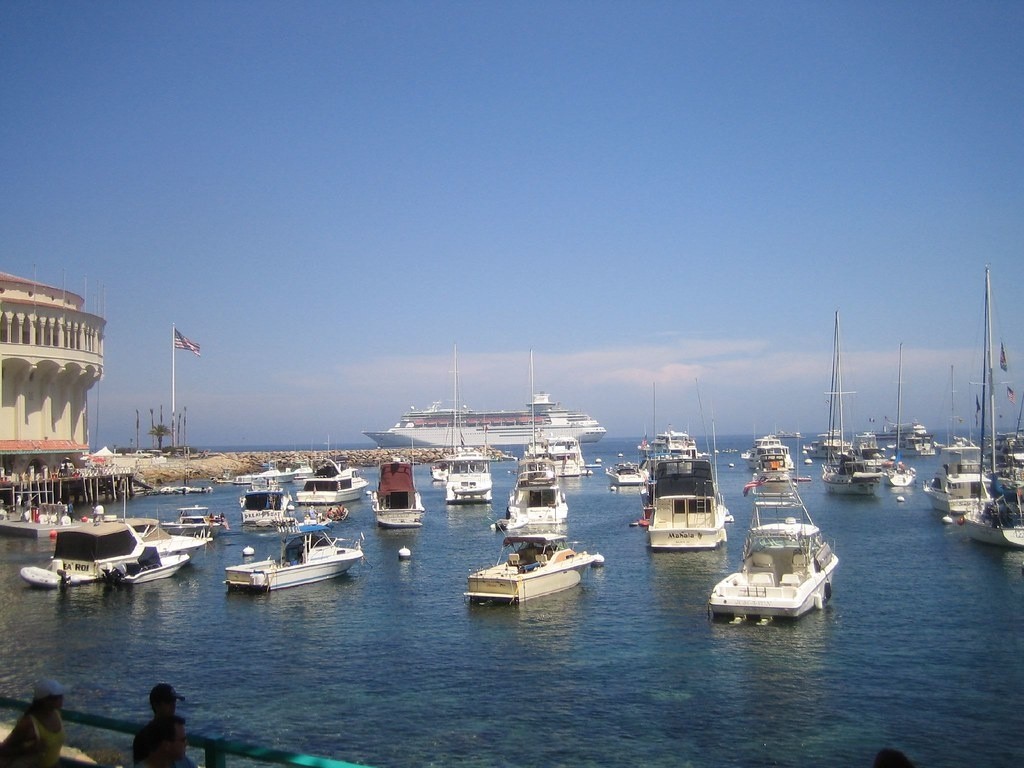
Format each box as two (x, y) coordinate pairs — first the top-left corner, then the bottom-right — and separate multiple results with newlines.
(132, 450), (154, 458)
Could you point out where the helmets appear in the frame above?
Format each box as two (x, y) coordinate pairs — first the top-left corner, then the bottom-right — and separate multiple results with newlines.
(35, 680), (63, 700)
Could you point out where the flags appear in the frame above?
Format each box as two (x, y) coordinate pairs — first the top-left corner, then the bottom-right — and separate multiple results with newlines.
(174, 328), (200, 358)
(975, 395), (980, 412)
(743, 476), (769, 497)
(642, 434), (648, 446)
(1007, 387), (1016, 404)
(1000, 343), (1007, 372)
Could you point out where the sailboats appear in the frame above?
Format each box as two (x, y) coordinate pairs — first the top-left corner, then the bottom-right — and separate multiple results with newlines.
(430, 344), (467, 480)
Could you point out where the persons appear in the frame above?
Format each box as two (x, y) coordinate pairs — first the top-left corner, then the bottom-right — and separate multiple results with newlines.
(208, 513), (231, 530)
(95, 503), (104, 521)
(134, 683), (185, 762)
(309, 504), (345, 523)
(69, 501), (74, 523)
(135, 716), (197, 768)
(0, 678), (66, 768)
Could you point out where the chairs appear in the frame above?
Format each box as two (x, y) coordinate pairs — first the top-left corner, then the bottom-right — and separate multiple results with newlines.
(792, 554), (806, 575)
(535, 554), (550, 565)
(749, 553), (778, 587)
(508, 553), (527, 566)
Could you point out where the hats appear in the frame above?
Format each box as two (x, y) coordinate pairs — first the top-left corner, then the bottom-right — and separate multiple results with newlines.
(150, 684), (185, 701)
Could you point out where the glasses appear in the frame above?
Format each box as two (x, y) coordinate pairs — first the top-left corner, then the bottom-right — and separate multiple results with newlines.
(174, 736), (188, 745)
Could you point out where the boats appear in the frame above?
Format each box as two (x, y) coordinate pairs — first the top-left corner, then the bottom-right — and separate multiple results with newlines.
(369, 460), (423, 523)
(708, 500), (840, 621)
(256, 517), (296, 527)
(444, 453), (493, 503)
(104, 547), (193, 584)
(239, 476), (290, 524)
(379, 521), (422, 528)
(53, 517), (212, 576)
(160, 486), (213, 494)
(233, 468), (295, 484)
(161, 506), (224, 537)
(327, 506), (350, 522)
(295, 453), (368, 505)
(464, 533), (595, 603)
(491, 434), (601, 531)
(362, 393), (606, 447)
(224, 525), (365, 591)
(605, 429), (734, 550)
(294, 464), (313, 479)
(414, 416), (544, 427)
(297, 512), (332, 527)
(19, 564), (81, 589)
(739, 263), (1024, 547)
